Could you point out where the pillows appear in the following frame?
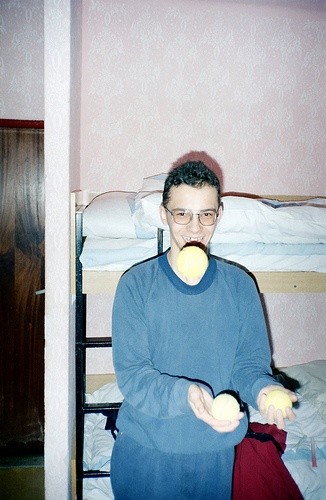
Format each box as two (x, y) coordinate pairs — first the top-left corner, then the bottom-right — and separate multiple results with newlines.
(82, 191), (137, 240)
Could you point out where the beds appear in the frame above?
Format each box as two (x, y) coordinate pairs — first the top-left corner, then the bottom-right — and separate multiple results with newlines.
(71, 191), (326, 294)
(71, 367), (289, 500)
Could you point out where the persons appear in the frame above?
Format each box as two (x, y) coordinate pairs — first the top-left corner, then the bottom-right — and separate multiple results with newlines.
(111, 160), (298, 500)
